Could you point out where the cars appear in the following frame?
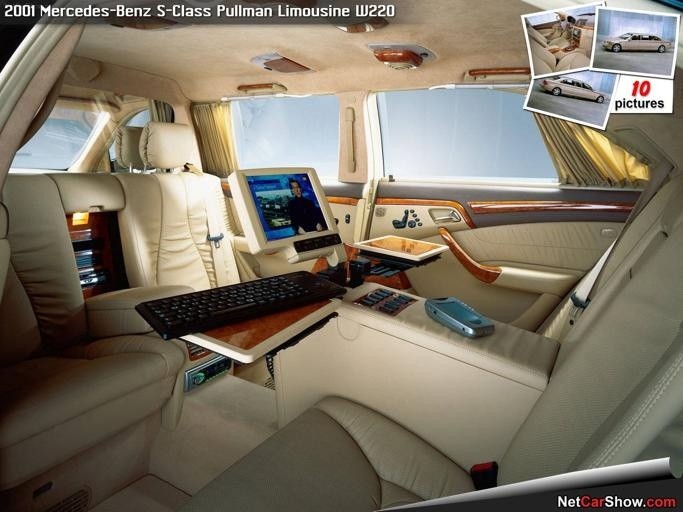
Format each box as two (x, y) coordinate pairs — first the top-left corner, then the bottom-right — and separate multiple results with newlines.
(536, 74), (606, 104)
(600, 31), (671, 54)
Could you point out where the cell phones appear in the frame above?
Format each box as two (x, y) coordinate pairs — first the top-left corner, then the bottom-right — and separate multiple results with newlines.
(425, 294), (496, 339)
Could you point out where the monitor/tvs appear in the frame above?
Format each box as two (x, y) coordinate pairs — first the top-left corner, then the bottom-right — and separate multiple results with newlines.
(227, 165), (340, 256)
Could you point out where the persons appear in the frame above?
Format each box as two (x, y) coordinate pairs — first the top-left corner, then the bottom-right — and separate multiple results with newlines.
(285, 178), (323, 235)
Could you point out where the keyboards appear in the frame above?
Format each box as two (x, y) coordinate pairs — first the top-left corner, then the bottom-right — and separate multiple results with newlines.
(132, 270), (347, 341)
